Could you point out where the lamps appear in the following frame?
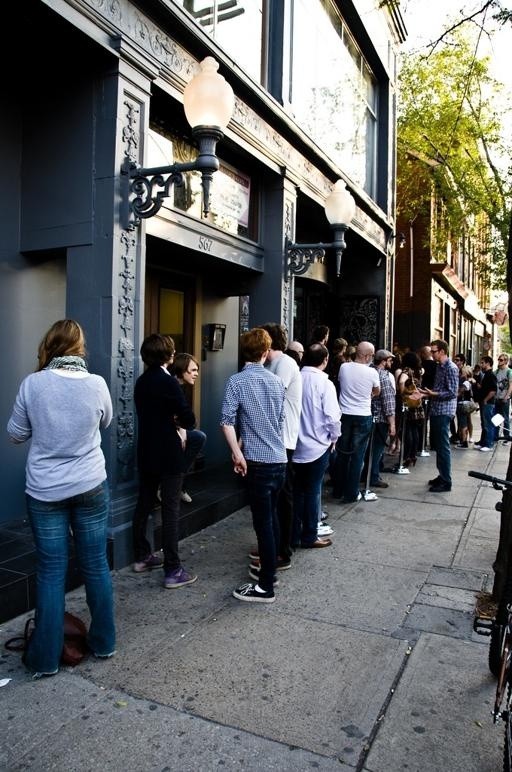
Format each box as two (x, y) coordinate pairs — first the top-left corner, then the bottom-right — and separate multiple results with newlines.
(120, 55), (236, 233)
(284, 178), (356, 284)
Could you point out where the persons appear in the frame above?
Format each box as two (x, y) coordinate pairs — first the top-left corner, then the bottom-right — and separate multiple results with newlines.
(230, 324), (302, 570)
(289, 339), (334, 536)
(156, 352), (206, 505)
(310, 325), (330, 346)
(132, 334), (198, 588)
(326, 339), (342, 397)
(455, 366), (470, 449)
(394, 352), (426, 468)
(291, 343), (332, 549)
(468, 366), (476, 442)
(474, 356), (498, 455)
(365, 349), (396, 489)
(6, 319), (118, 676)
(449, 354), (465, 442)
(473, 364), (484, 412)
(337, 337), (348, 357)
(220, 327), (288, 607)
(494, 352), (512, 446)
(334, 341), (380, 503)
(411, 339), (459, 492)
(421, 345), (437, 388)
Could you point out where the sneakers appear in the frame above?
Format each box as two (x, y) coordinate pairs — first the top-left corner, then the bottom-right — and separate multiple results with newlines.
(480, 446), (494, 451)
(133, 554), (164, 573)
(429, 486), (451, 492)
(457, 441), (469, 449)
(250, 557), (291, 571)
(181, 492), (192, 503)
(233, 584), (276, 602)
(250, 550), (293, 558)
(165, 568), (197, 588)
(248, 570), (279, 585)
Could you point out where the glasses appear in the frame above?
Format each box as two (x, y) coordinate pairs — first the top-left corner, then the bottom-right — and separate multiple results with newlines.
(498, 358), (504, 362)
(431, 350), (438, 354)
(455, 359), (460, 362)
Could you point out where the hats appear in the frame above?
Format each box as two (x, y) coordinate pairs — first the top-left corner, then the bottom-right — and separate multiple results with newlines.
(377, 350), (396, 359)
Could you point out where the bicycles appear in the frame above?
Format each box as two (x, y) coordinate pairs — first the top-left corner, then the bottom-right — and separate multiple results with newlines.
(468, 469), (512, 771)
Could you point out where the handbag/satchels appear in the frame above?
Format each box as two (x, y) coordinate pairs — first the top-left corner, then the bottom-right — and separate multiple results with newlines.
(458, 389), (476, 414)
(402, 379), (421, 407)
(5, 610), (88, 668)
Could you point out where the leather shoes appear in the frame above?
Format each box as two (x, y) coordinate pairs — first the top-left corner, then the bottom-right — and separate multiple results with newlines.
(313, 539), (332, 547)
